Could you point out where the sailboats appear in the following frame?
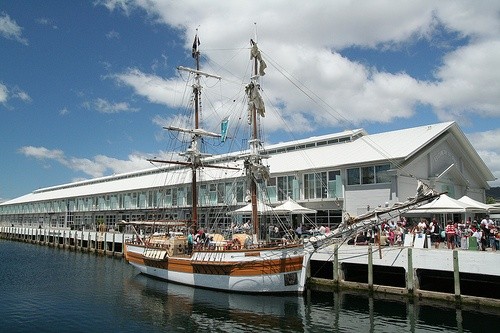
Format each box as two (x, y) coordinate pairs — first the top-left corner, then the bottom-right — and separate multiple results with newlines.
(119, 24), (449, 296)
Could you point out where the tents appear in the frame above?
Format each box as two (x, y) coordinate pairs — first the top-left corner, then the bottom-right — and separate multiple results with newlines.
(405, 192), (499, 224)
(224, 201), (271, 224)
(270, 197), (318, 230)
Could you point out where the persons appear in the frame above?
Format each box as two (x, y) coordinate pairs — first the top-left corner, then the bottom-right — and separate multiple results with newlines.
(188, 210), (500, 253)
